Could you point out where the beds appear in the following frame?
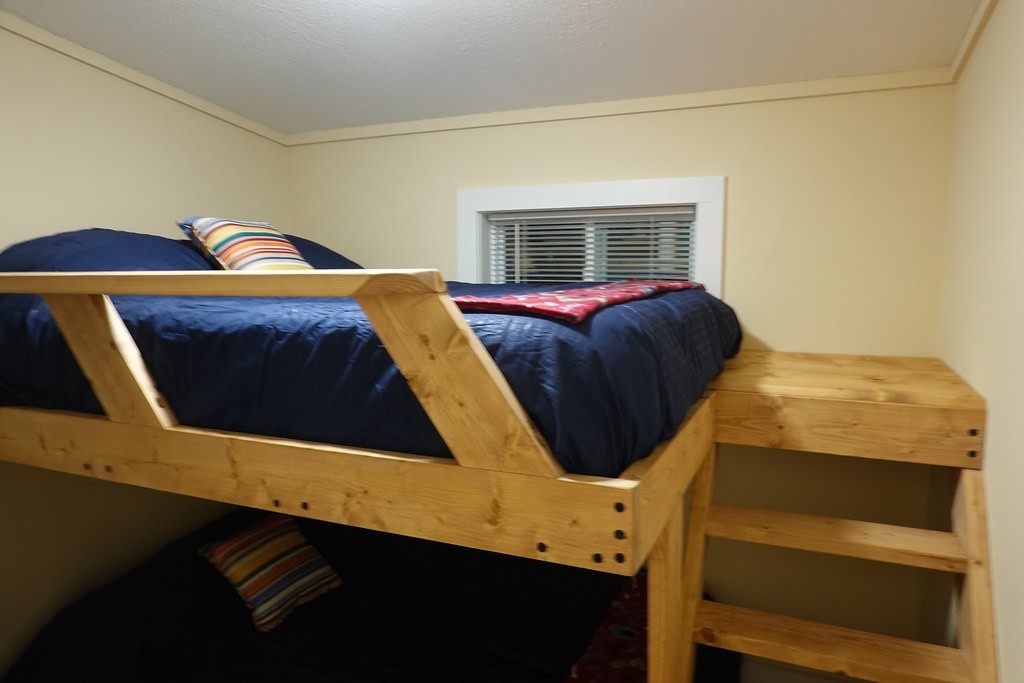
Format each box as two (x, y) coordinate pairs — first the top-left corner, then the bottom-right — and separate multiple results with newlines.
(0, 272), (998, 683)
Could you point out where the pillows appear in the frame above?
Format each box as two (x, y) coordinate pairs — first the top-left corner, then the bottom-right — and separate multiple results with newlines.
(186, 509), (342, 632)
(176, 217), (313, 271)
